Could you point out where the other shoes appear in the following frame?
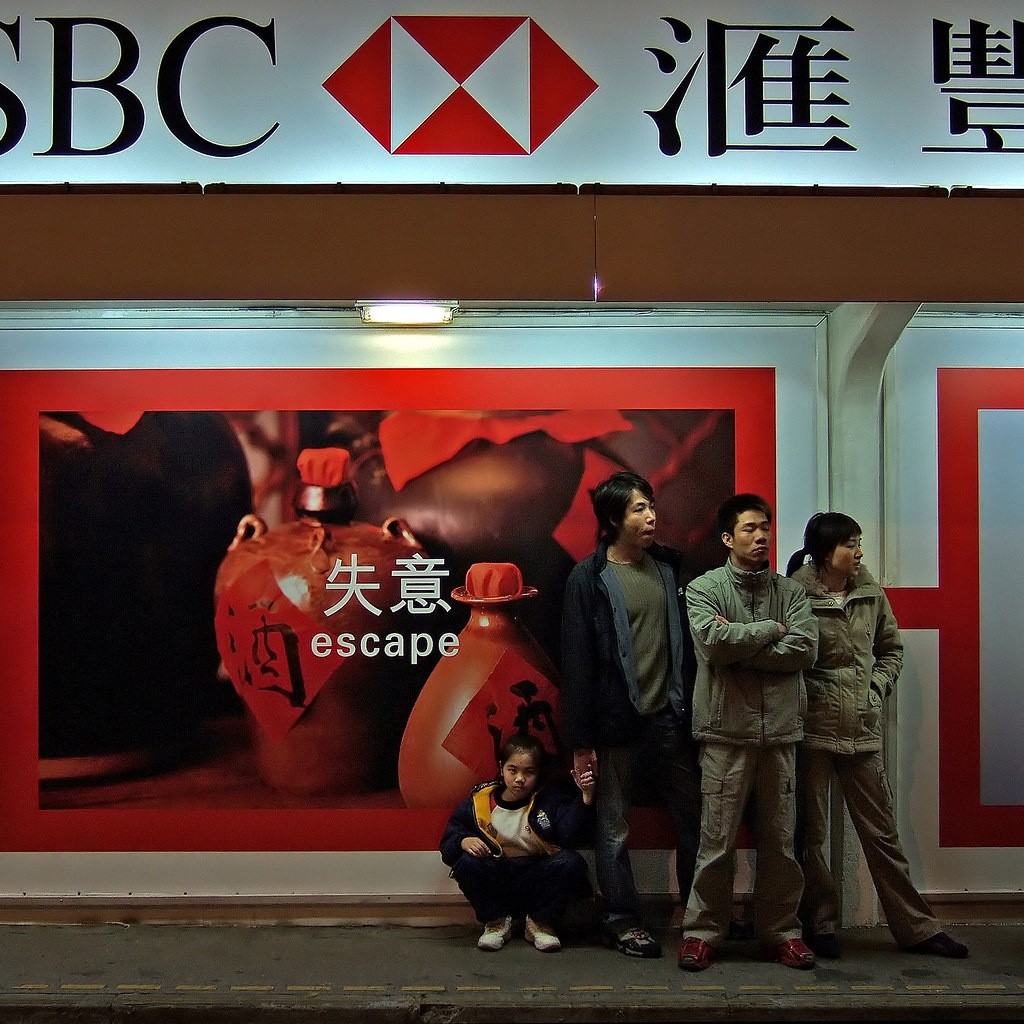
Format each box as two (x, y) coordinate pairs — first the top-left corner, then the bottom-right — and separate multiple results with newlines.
(809, 933), (839, 959)
(906, 932), (969, 959)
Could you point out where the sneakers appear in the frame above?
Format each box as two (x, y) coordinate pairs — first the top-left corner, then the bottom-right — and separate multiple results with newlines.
(772, 937), (815, 968)
(525, 914), (560, 952)
(681, 914), (749, 945)
(678, 937), (718, 970)
(603, 924), (661, 959)
(477, 915), (512, 950)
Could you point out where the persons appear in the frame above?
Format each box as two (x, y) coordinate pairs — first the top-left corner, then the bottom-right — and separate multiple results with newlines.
(678, 492), (820, 969)
(786, 512), (969, 957)
(561, 472), (702, 955)
(439, 735), (595, 950)
